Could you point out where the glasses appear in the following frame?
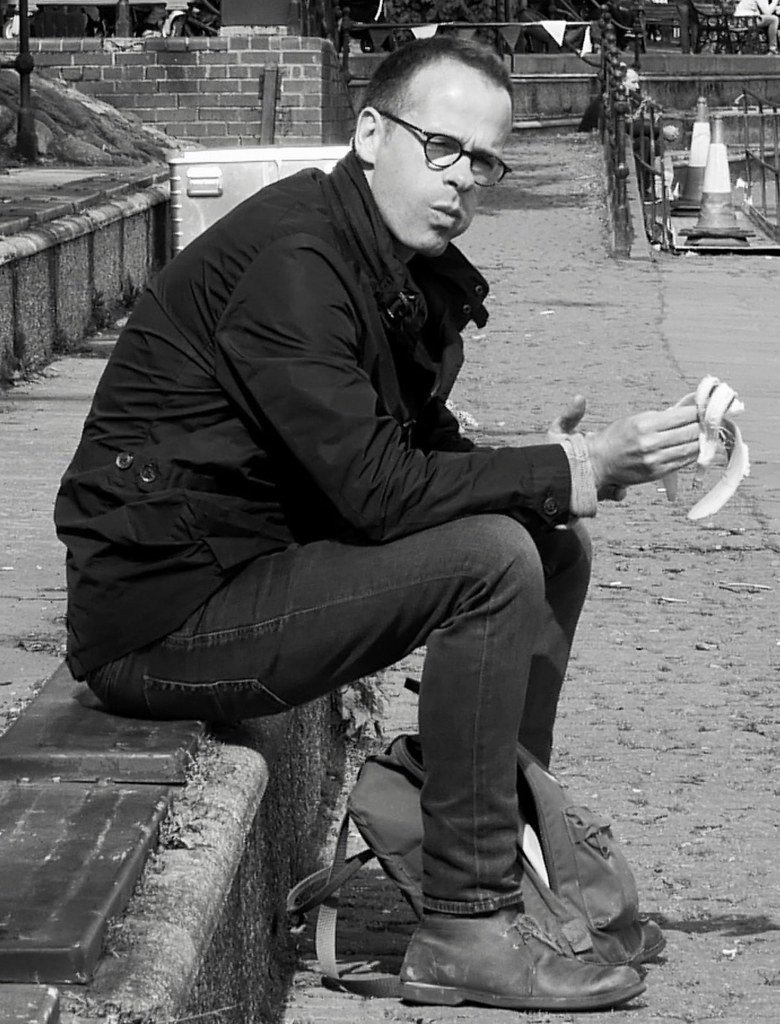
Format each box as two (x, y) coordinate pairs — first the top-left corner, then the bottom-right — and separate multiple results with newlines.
(379, 107), (514, 188)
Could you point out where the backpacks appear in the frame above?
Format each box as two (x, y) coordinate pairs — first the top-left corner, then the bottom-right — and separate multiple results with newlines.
(285, 677), (638, 998)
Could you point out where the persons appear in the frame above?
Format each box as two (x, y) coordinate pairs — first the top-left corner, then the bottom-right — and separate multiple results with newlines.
(734, 0), (780, 55)
(55, 35), (700, 1009)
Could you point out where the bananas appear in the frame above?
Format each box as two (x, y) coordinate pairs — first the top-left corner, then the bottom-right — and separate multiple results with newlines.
(660, 374), (751, 521)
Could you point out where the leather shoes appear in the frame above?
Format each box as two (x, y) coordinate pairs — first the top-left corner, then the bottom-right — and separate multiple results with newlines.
(638, 914), (666, 961)
(399, 914), (648, 1009)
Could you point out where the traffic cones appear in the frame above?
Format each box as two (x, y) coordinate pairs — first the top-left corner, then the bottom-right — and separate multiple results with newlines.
(676, 114), (756, 247)
(670, 95), (716, 219)
